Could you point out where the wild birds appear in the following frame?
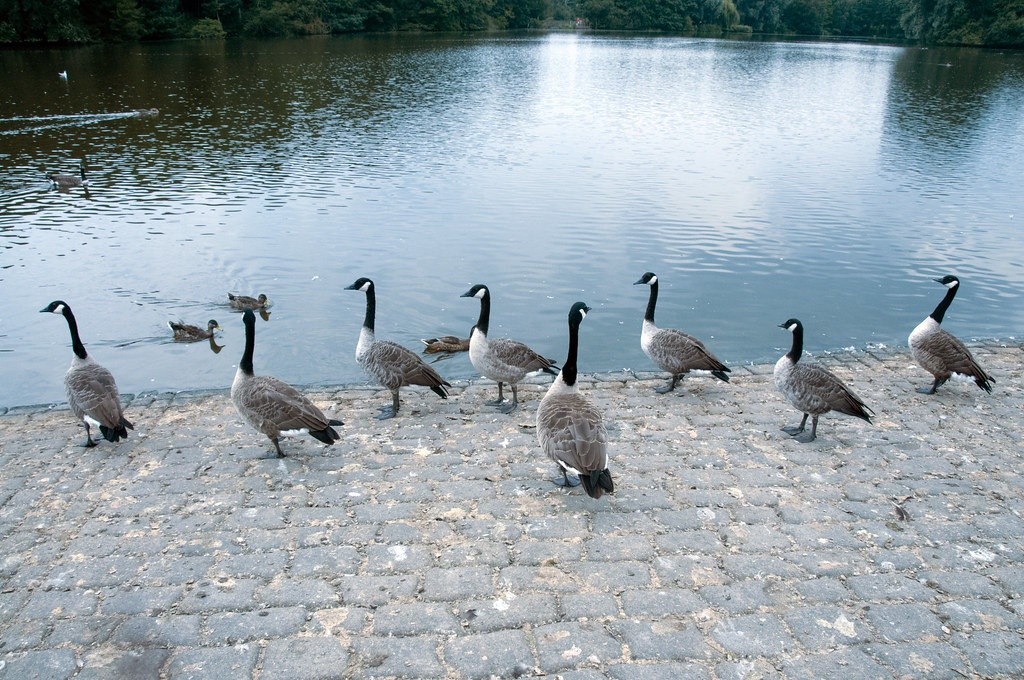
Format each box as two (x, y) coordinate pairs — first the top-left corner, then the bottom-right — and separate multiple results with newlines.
(227, 292), (272, 309)
(460, 284), (562, 415)
(59, 71), (67, 76)
(230, 309), (345, 459)
(130, 108), (159, 114)
(776, 318), (876, 443)
(39, 300), (134, 447)
(633, 271), (733, 395)
(420, 336), (470, 354)
(259, 308), (271, 321)
(45, 165), (92, 187)
(907, 274), (996, 397)
(209, 337), (226, 355)
(344, 277), (452, 420)
(535, 301), (615, 500)
(167, 319), (224, 340)
